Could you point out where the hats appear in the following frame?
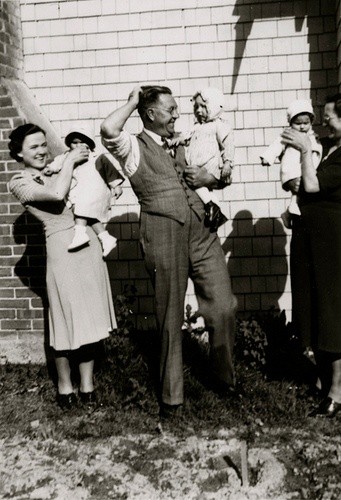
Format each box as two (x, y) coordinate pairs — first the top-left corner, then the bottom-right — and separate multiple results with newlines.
(287, 100), (316, 126)
(64, 128), (95, 150)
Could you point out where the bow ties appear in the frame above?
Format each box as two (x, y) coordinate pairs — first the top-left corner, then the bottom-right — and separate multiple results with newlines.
(159, 137), (175, 150)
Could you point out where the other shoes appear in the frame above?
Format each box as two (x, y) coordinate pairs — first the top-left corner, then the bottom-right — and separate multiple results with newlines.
(287, 202), (301, 216)
(204, 200), (220, 227)
(307, 397), (337, 419)
(55, 389), (78, 410)
(209, 210), (228, 234)
(220, 382), (245, 409)
(161, 403), (186, 421)
(102, 236), (117, 257)
(281, 211), (292, 230)
(69, 232), (90, 251)
(79, 388), (98, 410)
(298, 386), (323, 402)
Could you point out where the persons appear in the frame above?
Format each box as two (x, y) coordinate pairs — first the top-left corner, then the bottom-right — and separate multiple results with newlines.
(101, 84), (239, 427)
(41, 127), (125, 257)
(291, 93), (341, 418)
(259, 100), (323, 230)
(165, 87), (235, 233)
(9, 123), (118, 410)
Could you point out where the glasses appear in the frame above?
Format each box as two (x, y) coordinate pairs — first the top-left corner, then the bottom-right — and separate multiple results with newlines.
(148, 105), (178, 114)
(322, 115), (338, 124)
